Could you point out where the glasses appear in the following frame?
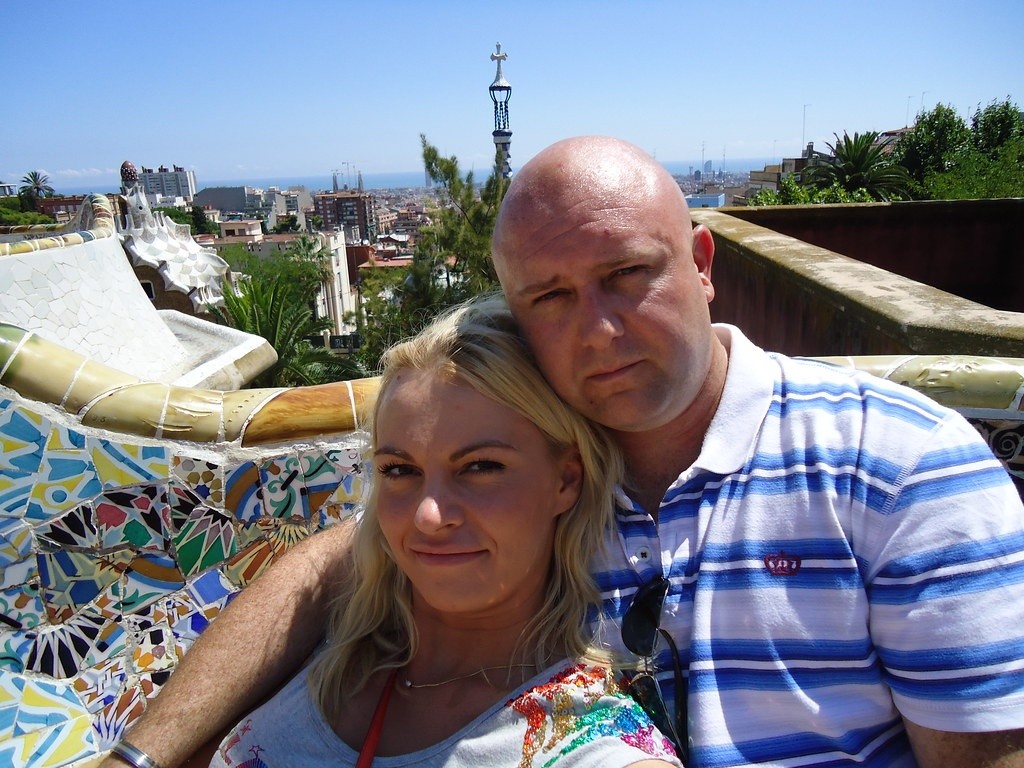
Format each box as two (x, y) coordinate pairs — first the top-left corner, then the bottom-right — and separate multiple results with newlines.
(621, 572), (690, 768)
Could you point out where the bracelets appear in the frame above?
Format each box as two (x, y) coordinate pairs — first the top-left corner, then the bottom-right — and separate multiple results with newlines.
(110, 738), (162, 768)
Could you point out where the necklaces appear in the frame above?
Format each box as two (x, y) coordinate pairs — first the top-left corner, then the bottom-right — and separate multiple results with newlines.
(403, 639), (559, 690)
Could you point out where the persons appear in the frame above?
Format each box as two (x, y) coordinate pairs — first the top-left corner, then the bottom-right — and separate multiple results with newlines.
(200, 295), (689, 768)
(96, 136), (1023, 768)
